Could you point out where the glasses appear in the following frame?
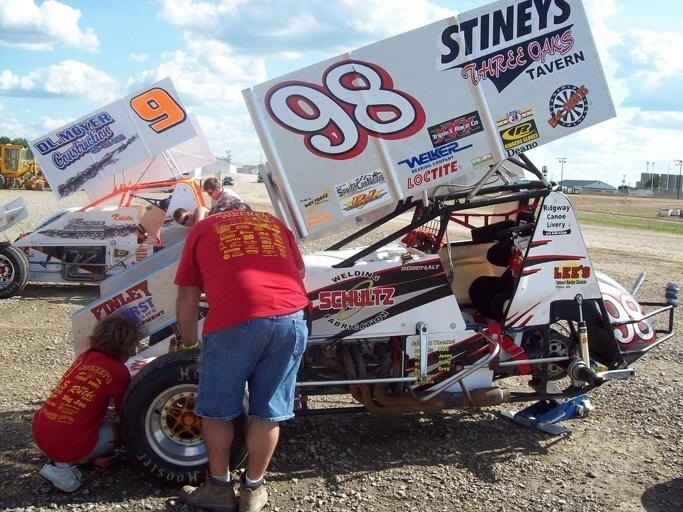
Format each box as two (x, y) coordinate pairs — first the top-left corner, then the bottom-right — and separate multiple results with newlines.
(179, 214), (189, 225)
(208, 188), (215, 196)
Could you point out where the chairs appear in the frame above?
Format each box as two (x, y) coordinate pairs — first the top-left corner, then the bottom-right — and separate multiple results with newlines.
(469, 210), (535, 321)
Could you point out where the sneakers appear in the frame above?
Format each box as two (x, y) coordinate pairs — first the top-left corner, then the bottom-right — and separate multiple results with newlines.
(39, 463), (81, 493)
(181, 470), (268, 512)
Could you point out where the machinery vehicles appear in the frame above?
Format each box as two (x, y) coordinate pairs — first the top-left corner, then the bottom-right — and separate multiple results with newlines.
(0, 143), (46, 190)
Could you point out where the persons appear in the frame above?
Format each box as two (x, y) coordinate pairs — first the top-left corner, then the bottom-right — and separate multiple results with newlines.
(172, 196), (313, 511)
(172, 206), (209, 226)
(31, 313), (139, 491)
(202, 177), (244, 219)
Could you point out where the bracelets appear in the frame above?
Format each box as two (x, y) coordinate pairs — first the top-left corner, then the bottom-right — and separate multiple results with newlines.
(182, 340), (198, 350)
